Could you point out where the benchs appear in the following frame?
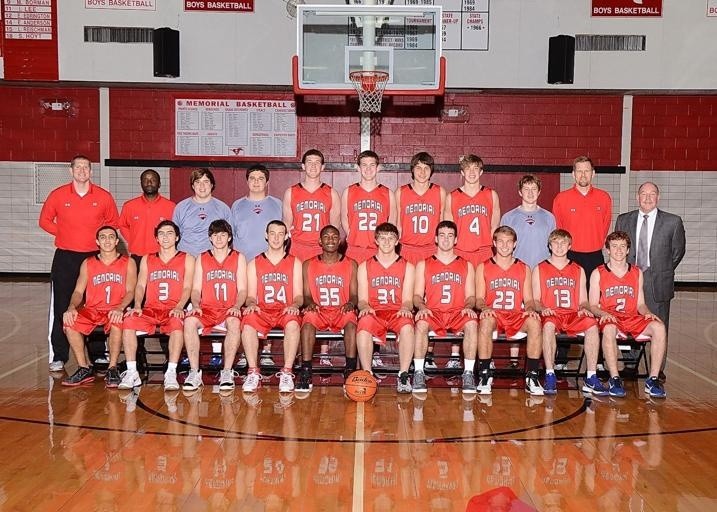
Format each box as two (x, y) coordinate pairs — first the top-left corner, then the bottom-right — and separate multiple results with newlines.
(84, 327), (653, 394)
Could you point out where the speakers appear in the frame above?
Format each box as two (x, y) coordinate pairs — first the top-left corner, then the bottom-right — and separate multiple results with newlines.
(153, 28), (179, 78)
(548, 36), (575, 84)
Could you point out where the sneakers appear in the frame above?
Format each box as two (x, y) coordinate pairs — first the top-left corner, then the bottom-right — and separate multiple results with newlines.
(444, 359), (461, 369)
(103, 367), (143, 390)
(372, 357), (386, 369)
(319, 358), (333, 367)
(236, 358), (247, 368)
(583, 377), (626, 398)
(208, 355), (223, 366)
(260, 355), (275, 366)
(396, 371), (429, 395)
(242, 369), (262, 394)
(216, 365), (237, 391)
(554, 364), (568, 371)
(181, 356), (190, 365)
(276, 368), (295, 393)
(646, 376), (666, 398)
(162, 369), (204, 391)
(295, 366), (313, 393)
(48, 360), (64, 372)
(596, 363), (605, 374)
(95, 358), (110, 364)
(62, 365), (97, 387)
(461, 369), (494, 395)
(424, 357), (438, 369)
(523, 372), (559, 395)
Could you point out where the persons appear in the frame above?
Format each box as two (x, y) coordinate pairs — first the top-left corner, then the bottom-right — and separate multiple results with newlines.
(614, 180), (684, 381)
(588, 232), (667, 399)
(531, 229), (611, 396)
(173, 149), (558, 397)
(120, 168), (178, 368)
(117, 221), (193, 389)
(61, 227), (137, 389)
(38, 155), (121, 371)
(552, 155), (613, 374)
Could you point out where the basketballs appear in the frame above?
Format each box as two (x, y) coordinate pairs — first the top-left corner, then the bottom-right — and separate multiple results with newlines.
(346, 371), (377, 402)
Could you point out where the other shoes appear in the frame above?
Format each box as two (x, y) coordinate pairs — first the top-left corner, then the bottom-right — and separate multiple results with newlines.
(618, 367), (639, 374)
(137, 355), (147, 367)
(162, 358), (170, 368)
(509, 358), (520, 368)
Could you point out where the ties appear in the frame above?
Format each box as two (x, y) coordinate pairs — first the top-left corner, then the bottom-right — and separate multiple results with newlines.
(638, 215), (649, 273)
